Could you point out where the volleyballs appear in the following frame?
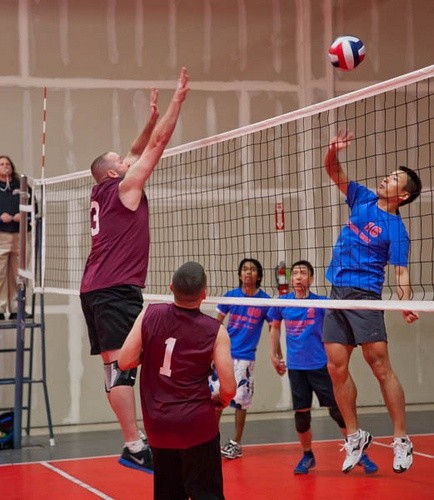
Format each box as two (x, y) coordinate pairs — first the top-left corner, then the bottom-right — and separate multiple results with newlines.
(330, 34), (366, 71)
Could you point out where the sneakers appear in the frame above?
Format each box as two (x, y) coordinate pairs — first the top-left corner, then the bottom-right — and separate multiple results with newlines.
(294, 454), (315, 474)
(393, 435), (414, 472)
(341, 429), (373, 473)
(357, 454), (377, 473)
(121, 437), (154, 472)
(221, 440), (243, 459)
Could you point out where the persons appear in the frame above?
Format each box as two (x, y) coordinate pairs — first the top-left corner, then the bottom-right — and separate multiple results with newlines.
(267, 261), (379, 475)
(322, 128), (422, 474)
(117, 260), (237, 500)
(0, 156), (39, 320)
(79, 67), (191, 475)
(207, 258), (286, 460)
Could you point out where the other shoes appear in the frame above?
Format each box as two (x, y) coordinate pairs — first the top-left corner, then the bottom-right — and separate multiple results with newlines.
(9, 311), (32, 319)
(0, 313), (4, 320)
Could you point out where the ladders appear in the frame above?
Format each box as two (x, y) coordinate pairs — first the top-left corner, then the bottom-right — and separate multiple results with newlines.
(0, 216), (59, 447)
